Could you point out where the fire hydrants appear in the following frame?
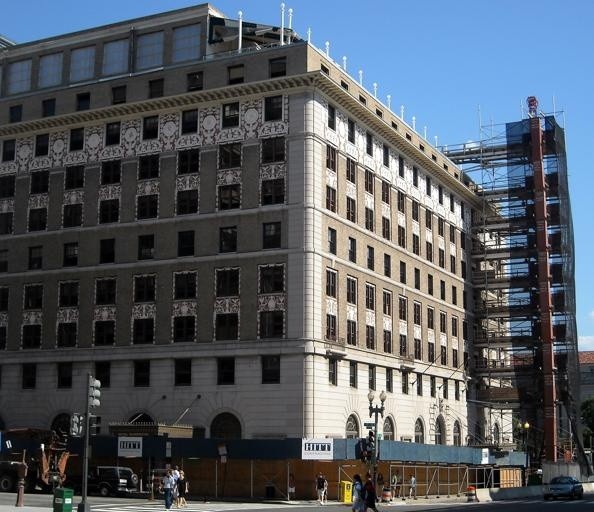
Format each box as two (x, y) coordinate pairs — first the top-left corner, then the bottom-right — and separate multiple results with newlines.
(15, 478), (25, 507)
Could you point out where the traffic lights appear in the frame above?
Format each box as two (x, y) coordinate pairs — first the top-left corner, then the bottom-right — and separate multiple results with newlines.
(88, 375), (101, 408)
(70, 413), (84, 437)
(369, 431), (375, 448)
(90, 415), (102, 435)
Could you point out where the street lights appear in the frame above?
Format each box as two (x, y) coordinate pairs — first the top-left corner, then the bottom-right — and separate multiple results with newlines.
(368, 390), (387, 501)
(517, 417), (529, 452)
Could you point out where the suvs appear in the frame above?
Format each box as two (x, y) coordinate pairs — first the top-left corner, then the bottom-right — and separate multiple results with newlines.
(66, 466), (138, 497)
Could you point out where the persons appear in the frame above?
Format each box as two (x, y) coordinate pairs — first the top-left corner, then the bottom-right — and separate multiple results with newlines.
(287, 473), (296, 500)
(375, 472), (386, 502)
(361, 471), (379, 512)
(387, 470), (397, 501)
(394, 469), (402, 498)
(350, 472), (364, 512)
(316, 470), (324, 504)
(321, 478), (328, 503)
(160, 464), (189, 509)
(407, 472), (418, 500)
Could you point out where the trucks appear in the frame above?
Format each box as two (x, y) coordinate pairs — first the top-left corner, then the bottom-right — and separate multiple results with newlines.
(0, 427), (69, 494)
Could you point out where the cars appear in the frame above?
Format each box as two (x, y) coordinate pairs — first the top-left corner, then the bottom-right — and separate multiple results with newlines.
(544, 476), (583, 501)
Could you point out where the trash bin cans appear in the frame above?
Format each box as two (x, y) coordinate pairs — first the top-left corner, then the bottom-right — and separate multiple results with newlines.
(265, 486), (275, 499)
(467, 487), (475, 502)
(53, 488), (73, 512)
(383, 488), (391, 503)
